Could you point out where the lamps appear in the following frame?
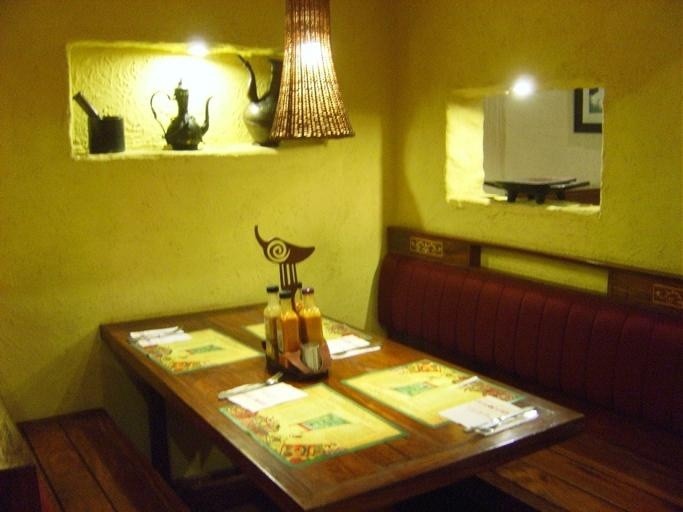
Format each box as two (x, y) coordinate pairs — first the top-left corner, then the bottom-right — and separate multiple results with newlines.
(269, 1), (356, 140)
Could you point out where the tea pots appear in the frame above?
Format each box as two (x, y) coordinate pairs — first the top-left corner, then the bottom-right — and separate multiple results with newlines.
(150, 54), (285, 151)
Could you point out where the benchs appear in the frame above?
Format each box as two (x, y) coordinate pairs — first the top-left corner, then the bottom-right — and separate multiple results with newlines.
(0, 396), (193, 512)
(380, 225), (682, 510)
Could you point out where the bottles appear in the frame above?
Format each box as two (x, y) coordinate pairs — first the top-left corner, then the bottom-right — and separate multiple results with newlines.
(263, 282), (323, 375)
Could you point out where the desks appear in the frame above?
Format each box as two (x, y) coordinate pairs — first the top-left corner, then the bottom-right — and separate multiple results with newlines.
(100, 299), (588, 510)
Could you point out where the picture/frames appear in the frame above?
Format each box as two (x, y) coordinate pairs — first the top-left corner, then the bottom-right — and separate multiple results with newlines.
(572, 88), (604, 132)
